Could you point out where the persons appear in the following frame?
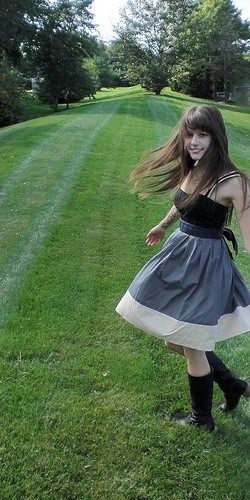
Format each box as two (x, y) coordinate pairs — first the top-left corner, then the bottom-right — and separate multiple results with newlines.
(115, 104), (250, 435)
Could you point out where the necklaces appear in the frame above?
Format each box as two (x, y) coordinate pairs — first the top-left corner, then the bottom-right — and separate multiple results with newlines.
(195, 171), (203, 185)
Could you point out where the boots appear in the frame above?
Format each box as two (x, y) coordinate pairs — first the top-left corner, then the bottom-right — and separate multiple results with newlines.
(172, 370), (218, 433)
(205, 351), (250, 413)
